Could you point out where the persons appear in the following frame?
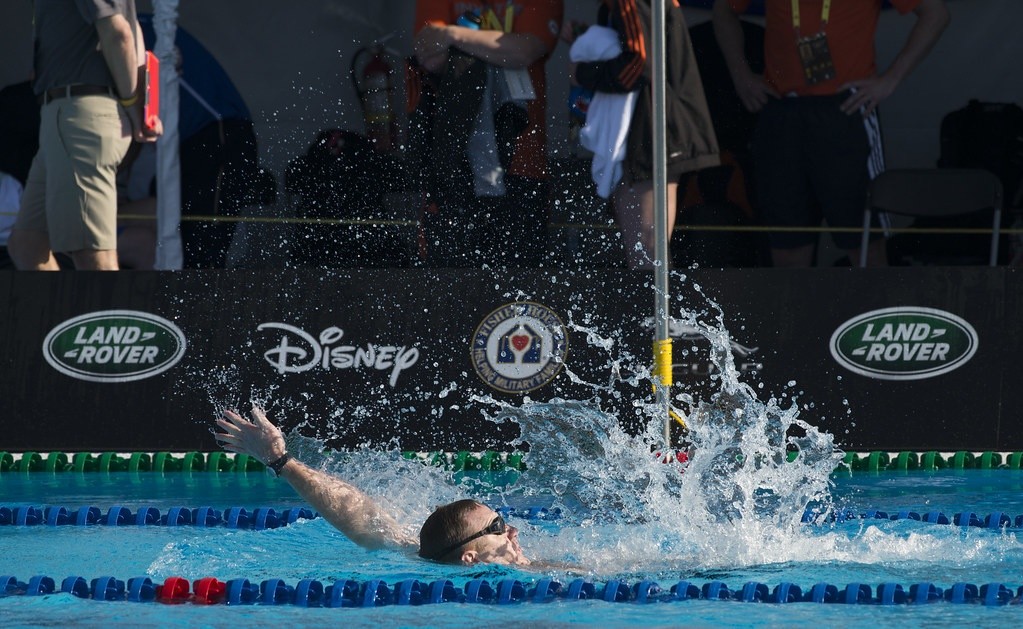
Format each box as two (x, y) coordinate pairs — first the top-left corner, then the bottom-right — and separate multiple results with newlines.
(6, 0), (164, 271)
(0, 173), (24, 246)
(566, 0), (722, 268)
(711, 0), (951, 267)
(414, 0), (564, 266)
(213, 405), (529, 566)
(138, 10), (259, 270)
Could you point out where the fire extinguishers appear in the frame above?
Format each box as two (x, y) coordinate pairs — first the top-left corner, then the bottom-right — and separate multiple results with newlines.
(358, 32), (402, 145)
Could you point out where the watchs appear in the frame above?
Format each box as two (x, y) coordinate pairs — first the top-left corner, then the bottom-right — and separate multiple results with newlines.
(265, 453), (293, 479)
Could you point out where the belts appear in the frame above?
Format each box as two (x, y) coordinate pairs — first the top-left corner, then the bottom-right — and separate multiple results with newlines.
(39, 85), (118, 105)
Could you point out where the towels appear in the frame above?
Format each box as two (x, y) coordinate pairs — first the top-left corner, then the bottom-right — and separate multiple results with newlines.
(569, 25), (641, 198)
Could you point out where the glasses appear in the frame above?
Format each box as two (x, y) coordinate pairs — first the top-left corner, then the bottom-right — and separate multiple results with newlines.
(419, 515), (505, 561)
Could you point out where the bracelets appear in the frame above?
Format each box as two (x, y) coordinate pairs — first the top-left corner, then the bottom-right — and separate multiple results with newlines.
(120, 96), (138, 107)
